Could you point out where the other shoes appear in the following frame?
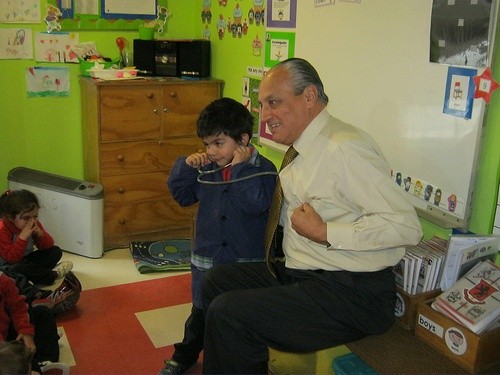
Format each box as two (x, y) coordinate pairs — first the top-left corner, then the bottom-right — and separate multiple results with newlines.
(37, 361), (70, 375)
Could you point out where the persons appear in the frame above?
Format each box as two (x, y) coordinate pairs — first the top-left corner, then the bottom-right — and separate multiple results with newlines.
(0, 190), (74, 375)
(202, 58), (425, 375)
(158, 98), (278, 375)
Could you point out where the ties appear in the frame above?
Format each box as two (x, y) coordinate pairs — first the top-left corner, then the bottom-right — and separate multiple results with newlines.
(266, 144), (297, 278)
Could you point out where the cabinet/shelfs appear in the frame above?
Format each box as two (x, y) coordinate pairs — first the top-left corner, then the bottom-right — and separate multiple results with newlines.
(80, 77), (224, 248)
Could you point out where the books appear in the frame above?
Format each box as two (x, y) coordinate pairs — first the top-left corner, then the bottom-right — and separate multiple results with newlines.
(396, 235), (500, 335)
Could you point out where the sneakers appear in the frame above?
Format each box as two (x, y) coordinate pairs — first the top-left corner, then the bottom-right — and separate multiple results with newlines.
(53, 260), (74, 278)
(162, 358), (196, 375)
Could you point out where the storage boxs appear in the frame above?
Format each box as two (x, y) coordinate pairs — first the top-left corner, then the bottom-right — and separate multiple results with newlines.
(397, 289), (500, 375)
